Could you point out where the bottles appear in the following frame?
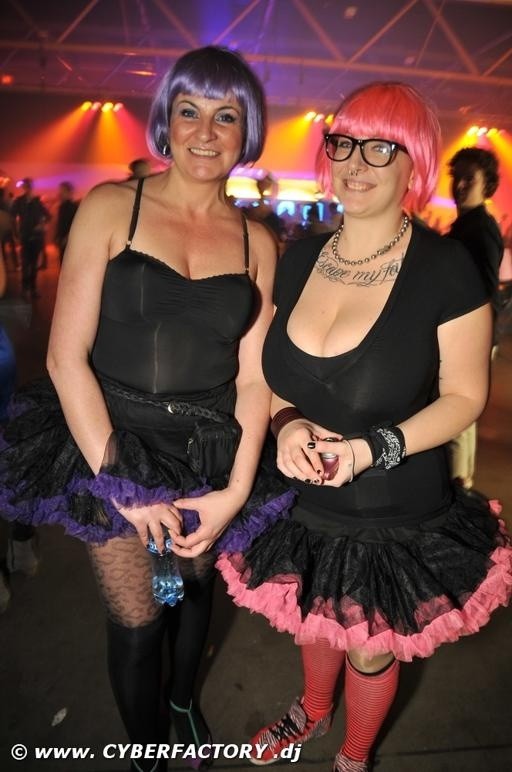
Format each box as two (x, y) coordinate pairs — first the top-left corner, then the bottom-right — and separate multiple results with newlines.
(147, 532), (187, 607)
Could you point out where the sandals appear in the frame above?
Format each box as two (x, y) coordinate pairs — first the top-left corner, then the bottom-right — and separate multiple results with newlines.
(169, 696), (215, 772)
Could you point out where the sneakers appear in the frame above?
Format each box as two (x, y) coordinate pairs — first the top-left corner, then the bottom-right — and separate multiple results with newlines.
(246, 692), (334, 766)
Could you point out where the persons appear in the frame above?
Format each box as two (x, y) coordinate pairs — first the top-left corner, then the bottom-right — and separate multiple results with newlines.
(0, 46), (512, 772)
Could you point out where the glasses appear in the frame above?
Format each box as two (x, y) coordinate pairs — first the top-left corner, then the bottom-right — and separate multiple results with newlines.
(323, 130), (409, 167)
